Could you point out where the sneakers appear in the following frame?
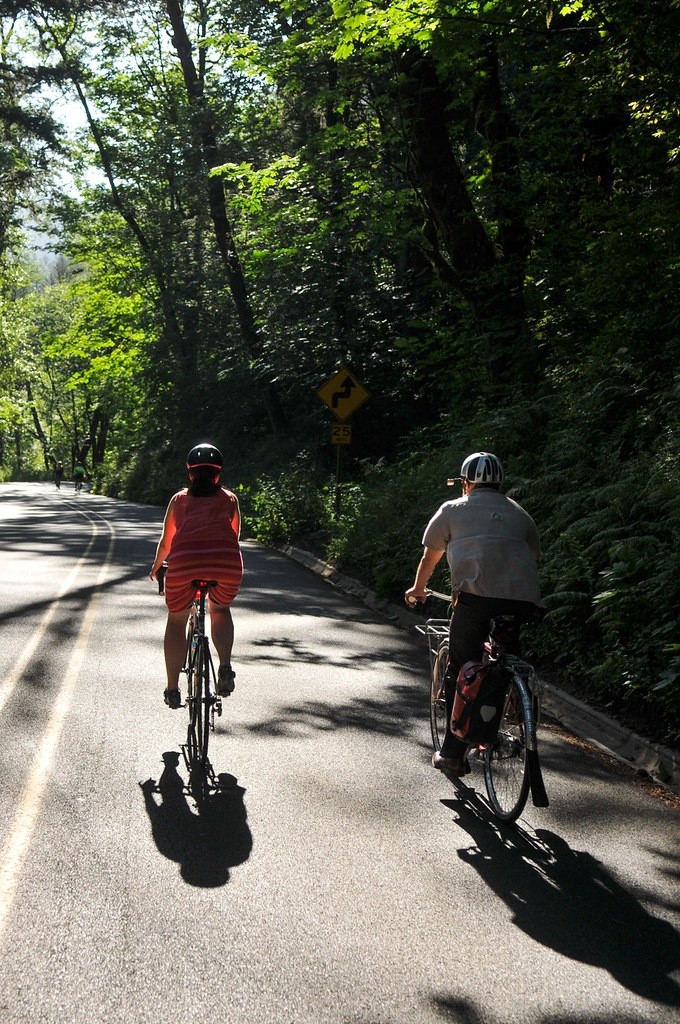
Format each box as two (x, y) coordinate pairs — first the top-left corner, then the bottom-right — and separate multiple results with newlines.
(217, 665), (234, 699)
(164, 688), (179, 704)
(432, 749), (468, 778)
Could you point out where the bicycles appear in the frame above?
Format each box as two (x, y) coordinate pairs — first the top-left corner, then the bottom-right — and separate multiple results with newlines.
(408, 590), (540, 823)
(73, 475), (82, 496)
(56, 476), (62, 490)
(151, 564), (233, 772)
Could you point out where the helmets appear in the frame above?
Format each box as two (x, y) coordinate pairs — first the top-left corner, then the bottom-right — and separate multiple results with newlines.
(186, 441), (224, 473)
(458, 451), (504, 486)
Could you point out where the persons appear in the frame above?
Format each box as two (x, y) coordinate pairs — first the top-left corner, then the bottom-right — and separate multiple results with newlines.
(149, 444), (244, 710)
(74, 461), (84, 491)
(56, 461), (62, 475)
(405, 452), (542, 777)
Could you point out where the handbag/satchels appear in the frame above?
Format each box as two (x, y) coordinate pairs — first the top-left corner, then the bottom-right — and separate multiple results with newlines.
(449, 657), (504, 742)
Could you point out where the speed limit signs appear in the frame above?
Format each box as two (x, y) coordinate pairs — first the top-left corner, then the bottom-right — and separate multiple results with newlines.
(331, 425), (352, 445)
